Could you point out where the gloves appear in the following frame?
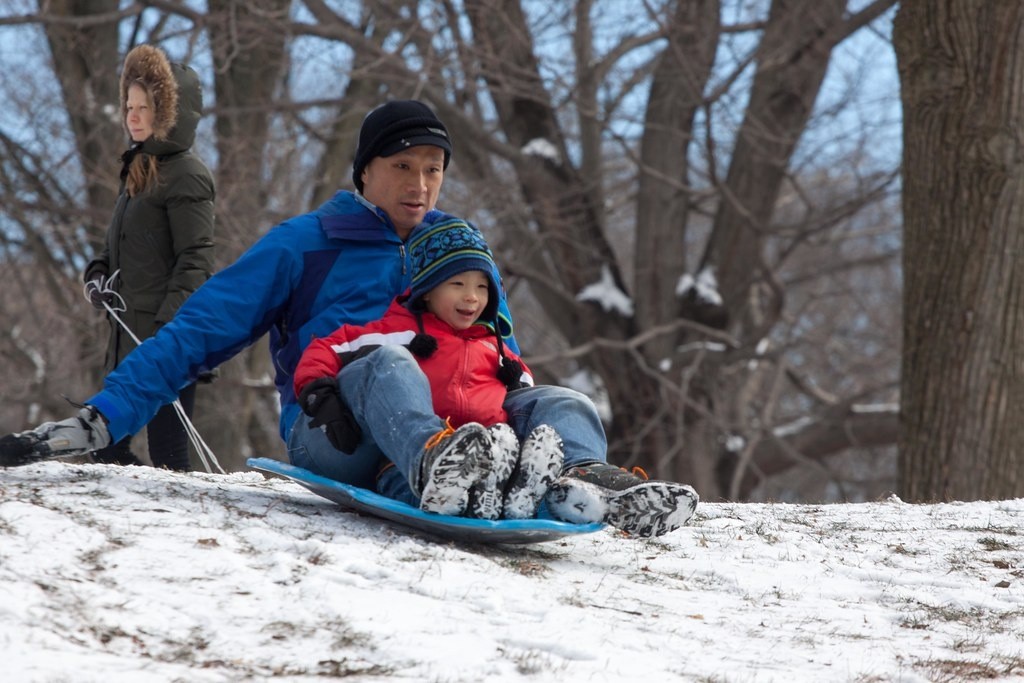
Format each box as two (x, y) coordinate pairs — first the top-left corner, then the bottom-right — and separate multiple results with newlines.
(86, 272), (115, 311)
(0, 406), (110, 465)
(297, 376), (365, 455)
(196, 374), (214, 385)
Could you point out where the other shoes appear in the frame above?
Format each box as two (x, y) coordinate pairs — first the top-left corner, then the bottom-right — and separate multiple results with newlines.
(503, 424), (564, 520)
(471, 424), (520, 519)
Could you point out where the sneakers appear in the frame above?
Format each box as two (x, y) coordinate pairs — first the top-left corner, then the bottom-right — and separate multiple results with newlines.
(419, 415), (494, 516)
(545, 462), (700, 540)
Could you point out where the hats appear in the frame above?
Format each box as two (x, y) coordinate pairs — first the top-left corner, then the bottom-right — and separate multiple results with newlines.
(352, 100), (452, 195)
(400, 210), (523, 386)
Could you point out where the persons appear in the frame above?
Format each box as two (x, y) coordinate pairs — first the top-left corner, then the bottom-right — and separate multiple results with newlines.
(82, 41), (217, 470)
(288, 211), (566, 520)
(0, 102), (698, 539)
